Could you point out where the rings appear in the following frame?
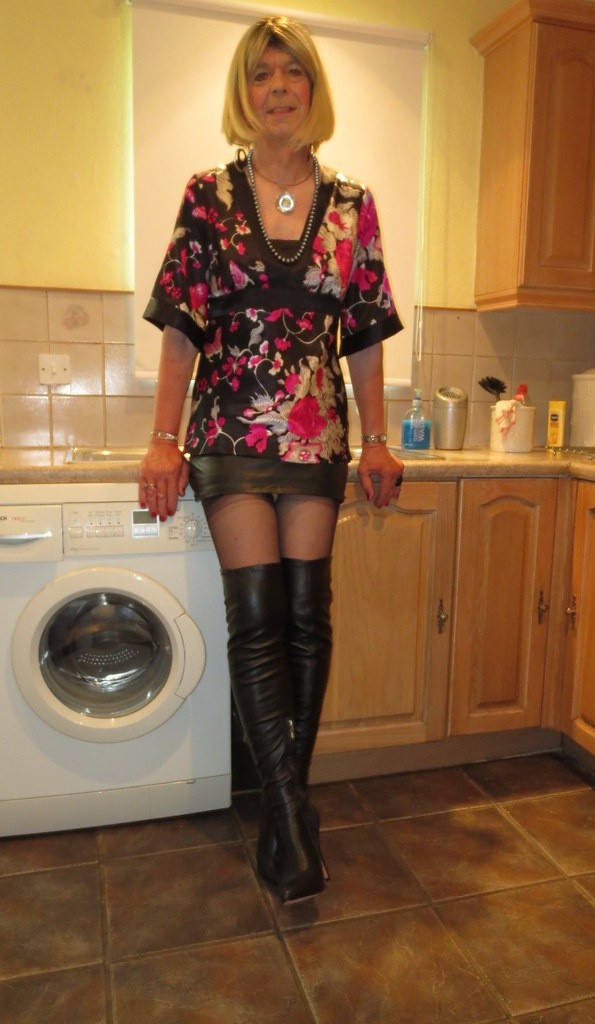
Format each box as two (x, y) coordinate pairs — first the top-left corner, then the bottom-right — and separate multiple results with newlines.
(145, 483), (157, 489)
(394, 476), (403, 486)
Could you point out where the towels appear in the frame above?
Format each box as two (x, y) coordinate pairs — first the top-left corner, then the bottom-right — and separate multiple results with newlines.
(496, 399), (524, 441)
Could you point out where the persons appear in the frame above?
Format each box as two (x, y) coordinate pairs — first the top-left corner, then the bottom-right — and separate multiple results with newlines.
(138, 17), (405, 905)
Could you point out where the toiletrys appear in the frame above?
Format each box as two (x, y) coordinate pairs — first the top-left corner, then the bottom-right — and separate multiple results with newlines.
(512, 383), (531, 407)
(401, 387), (430, 450)
(547, 400), (567, 447)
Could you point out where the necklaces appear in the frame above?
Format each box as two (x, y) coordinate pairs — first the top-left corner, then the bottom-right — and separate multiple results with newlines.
(246, 149), (319, 263)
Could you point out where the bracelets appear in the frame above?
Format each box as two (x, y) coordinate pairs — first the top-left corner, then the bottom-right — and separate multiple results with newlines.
(150, 431), (178, 440)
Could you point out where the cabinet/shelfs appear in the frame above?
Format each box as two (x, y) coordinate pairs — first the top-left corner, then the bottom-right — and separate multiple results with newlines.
(306, 477), (595, 784)
(469, 0), (595, 312)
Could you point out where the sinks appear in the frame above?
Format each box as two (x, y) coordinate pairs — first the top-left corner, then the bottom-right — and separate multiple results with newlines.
(66, 443), (445, 463)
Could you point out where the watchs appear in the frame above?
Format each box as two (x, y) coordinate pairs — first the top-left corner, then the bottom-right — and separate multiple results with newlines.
(362, 433), (388, 443)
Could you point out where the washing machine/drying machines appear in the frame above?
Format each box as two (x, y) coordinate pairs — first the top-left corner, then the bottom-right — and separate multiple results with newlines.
(2, 483), (232, 839)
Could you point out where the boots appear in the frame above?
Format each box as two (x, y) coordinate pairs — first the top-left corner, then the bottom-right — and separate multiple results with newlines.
(220, 555), (330, 904)
